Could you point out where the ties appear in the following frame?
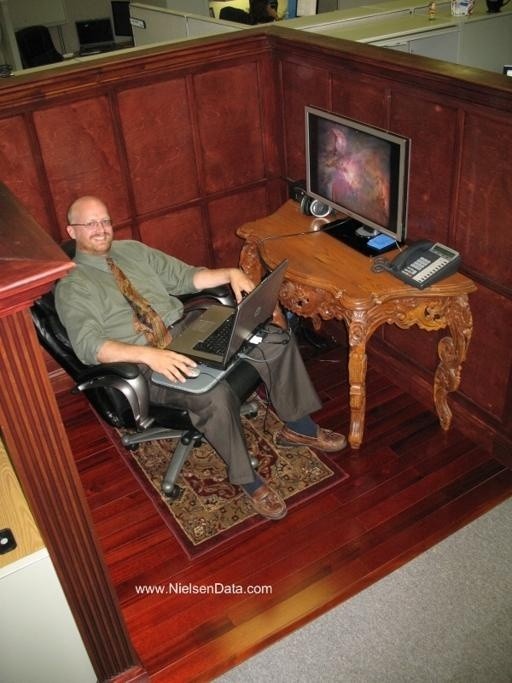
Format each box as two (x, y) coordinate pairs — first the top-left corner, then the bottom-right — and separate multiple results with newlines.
(105, 256), (173, 348)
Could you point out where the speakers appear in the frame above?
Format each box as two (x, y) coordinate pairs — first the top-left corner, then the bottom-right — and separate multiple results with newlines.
(309, 198), (333, 218)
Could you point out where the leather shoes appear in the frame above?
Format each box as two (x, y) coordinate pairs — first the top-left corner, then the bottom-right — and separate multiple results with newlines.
(280, 422), (348, 452)
(239, 469), (288, 519)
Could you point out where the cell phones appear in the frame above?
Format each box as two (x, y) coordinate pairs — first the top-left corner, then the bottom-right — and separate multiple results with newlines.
(364, 232), (398, 252)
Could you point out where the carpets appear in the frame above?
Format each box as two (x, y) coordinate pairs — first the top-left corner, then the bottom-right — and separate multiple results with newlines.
(85, 376), (350, 561)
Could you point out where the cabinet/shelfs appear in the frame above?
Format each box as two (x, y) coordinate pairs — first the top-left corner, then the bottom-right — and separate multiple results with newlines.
(368, 25), (461, 65)
(460, 14), (512, 78)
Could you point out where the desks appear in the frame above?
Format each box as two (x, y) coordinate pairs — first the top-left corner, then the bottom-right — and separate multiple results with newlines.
(235, 199), (478, 449)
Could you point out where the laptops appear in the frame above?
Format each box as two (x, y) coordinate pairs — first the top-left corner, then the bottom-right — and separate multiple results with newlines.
(163, 260), (286, 369)
(75, 17), (116, 56)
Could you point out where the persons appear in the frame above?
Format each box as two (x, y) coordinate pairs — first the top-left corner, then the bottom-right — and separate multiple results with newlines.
(52, 193), (347, 520)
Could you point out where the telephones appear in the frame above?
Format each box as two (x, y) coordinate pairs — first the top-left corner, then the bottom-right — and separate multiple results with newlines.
(386, 238), (461, 290)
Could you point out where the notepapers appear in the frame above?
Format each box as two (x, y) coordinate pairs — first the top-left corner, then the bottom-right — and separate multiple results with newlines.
(367, 233), (395, 251)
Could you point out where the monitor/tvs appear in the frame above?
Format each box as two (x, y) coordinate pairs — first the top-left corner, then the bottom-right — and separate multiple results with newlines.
(303, 105), (412, 257)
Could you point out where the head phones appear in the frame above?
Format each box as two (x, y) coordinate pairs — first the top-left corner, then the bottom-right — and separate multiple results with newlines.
(289, 179), (313, 217)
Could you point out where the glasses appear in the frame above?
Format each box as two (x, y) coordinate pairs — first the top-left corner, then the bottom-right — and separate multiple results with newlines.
(70, 218), (112, 229)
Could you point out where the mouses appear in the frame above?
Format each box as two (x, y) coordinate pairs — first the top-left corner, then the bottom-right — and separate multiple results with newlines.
(178, 366), (200, 378)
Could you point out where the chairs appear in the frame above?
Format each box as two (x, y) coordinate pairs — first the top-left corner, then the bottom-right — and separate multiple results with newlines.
(28, 277), (260, 498)
(14, 25), (64, 69)
(219, 6), (257, 26)
